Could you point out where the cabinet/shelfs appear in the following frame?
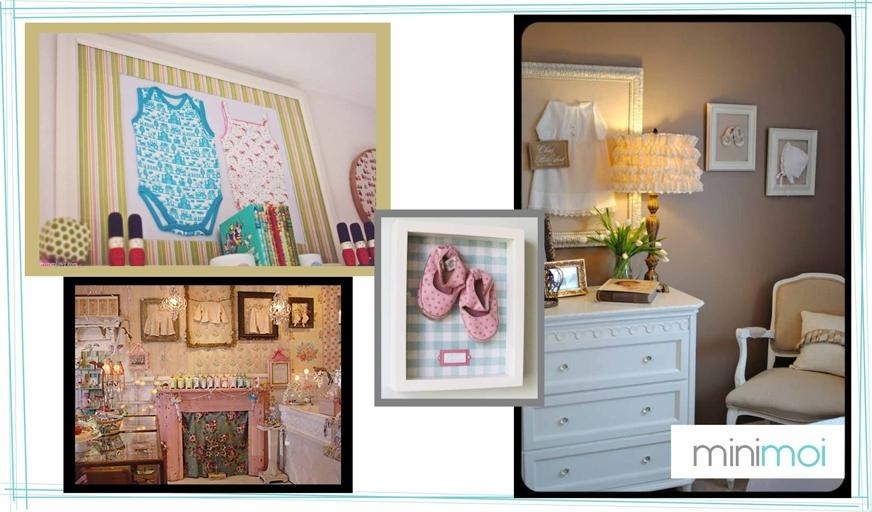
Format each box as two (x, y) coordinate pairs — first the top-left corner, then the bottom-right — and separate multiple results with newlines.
(521, 285), (706, 493)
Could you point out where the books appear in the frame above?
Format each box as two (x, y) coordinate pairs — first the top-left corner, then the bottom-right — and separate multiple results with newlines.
(594, 277), (660, 305)
(217, 205), (300, 265)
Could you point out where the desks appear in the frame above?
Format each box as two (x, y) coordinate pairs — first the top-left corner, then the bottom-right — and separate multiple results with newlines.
(76, 414), (165, 485)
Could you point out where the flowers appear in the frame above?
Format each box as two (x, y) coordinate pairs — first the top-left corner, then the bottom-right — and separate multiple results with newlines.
(587, 205), (669, 263)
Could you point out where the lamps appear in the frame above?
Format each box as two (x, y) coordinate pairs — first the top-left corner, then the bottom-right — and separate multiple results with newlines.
(304, 368), (310, 384)
(611, 128), (703, 293)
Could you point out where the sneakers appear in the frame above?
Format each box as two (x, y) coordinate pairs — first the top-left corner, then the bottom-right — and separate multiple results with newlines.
(723, 127), (744, 147)
(416, 244), (500, 343)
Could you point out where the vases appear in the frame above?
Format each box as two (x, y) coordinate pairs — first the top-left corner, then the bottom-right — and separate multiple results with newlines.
(612, 252), (633, 280)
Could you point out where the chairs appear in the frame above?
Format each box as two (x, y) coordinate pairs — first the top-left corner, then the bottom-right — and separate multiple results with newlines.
(721, 272), (847, 487)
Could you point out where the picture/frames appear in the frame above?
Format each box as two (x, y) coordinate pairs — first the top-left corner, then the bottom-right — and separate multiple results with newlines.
(705, 101), (756, 173)
(128, 351), (146, 367)
(75, 293), (121, 317)
(56, 34), (345, 268)
(391, 222), (526, 392)
(521, 62), (644, 249)
(237, 291), (279, 340)
(289, 297), (315, 328)
(541, 258), (587, 299)
(766, 127), (817, 196)
(268, 359), (292, 388)
(184, 285), (236, 351)
(140, 298), (180, 342)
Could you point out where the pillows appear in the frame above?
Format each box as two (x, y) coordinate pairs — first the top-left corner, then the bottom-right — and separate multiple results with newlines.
(797, 346), (843, 375)
(789, 310), (845, 372)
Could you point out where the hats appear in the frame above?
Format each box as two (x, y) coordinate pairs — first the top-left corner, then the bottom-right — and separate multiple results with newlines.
(780, 142), (809, 182)
